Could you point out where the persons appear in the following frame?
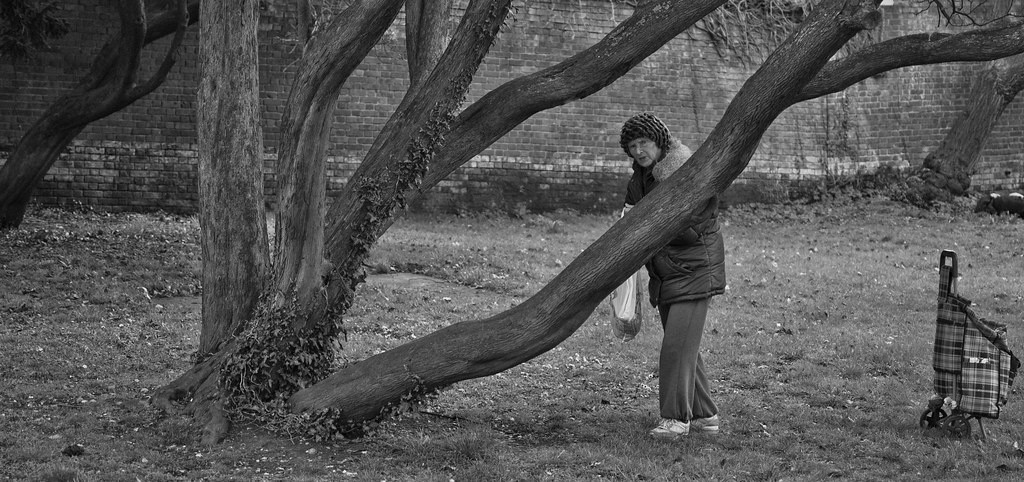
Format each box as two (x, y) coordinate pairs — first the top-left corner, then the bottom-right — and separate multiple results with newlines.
(621, 116), (728, 436)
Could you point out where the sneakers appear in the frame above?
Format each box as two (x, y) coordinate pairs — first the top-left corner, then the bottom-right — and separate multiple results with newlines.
(690, 413), (719, 434)
(650, 418), (690, 437)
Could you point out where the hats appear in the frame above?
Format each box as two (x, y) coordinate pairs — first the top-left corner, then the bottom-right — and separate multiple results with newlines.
(619, 113), (671, 158)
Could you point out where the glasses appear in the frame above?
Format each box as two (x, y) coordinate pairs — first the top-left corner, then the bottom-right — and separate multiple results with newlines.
(629, 139), (653, 150)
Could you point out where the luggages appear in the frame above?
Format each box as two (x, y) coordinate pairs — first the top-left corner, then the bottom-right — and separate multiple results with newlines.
(919, 249), (1021, 439)
